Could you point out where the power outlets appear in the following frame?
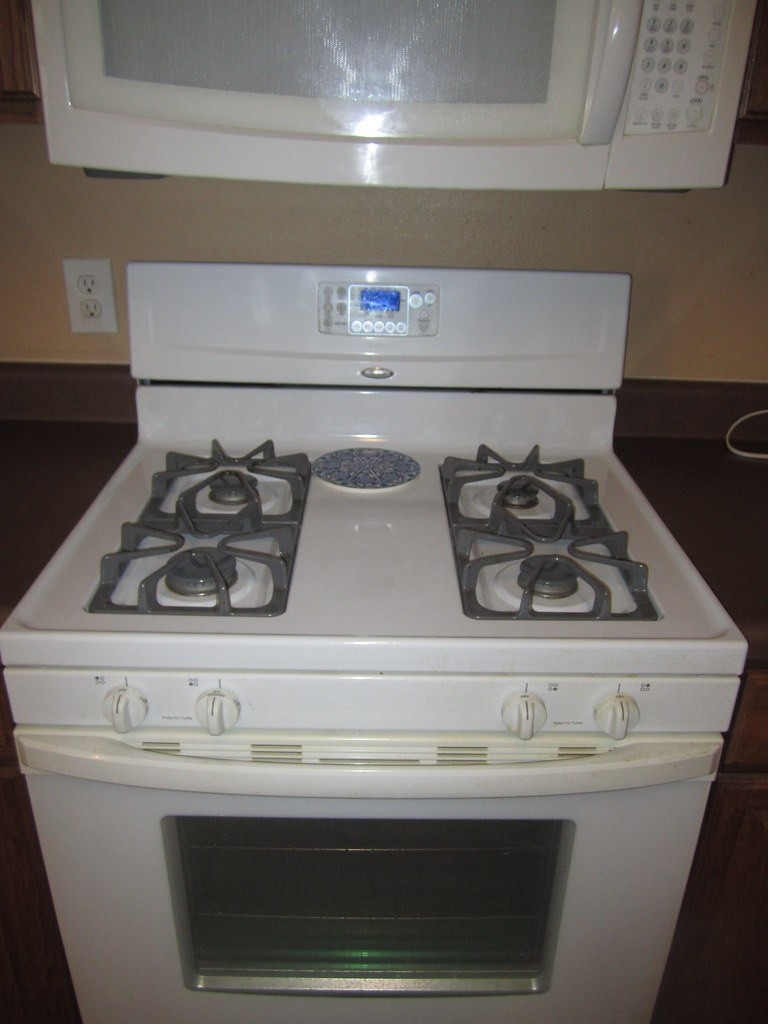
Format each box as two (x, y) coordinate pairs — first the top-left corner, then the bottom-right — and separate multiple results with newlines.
(62, 257), (119, 334)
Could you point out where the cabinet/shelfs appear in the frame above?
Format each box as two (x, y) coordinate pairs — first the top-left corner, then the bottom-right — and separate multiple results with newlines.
(652, 666), (768, 1024)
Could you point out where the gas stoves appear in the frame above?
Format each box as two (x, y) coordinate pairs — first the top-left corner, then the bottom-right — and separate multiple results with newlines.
(0, 262), (747, 732)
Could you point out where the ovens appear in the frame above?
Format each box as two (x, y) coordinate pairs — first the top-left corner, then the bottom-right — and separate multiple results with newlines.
(14, 736), (720, 1024)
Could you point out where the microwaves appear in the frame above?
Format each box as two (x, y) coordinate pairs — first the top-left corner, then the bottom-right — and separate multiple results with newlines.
(30, 0), (754, 192)
